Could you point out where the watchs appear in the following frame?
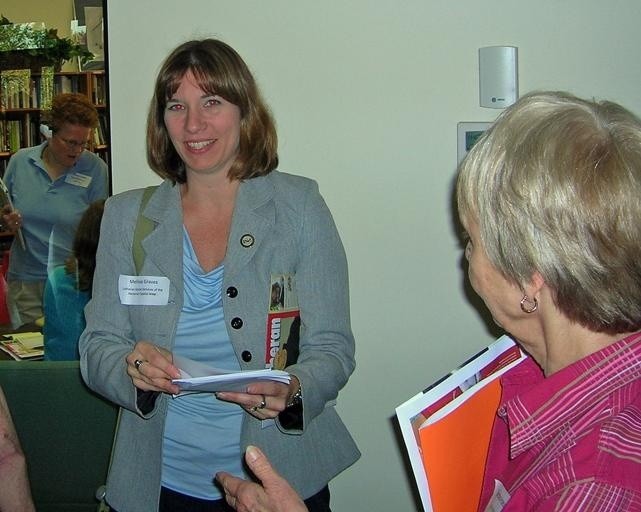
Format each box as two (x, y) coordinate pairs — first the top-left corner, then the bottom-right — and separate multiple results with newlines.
(285, 382), (305, 412)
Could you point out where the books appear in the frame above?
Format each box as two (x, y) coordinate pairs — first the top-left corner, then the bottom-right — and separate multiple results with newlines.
(1, 56), (107, 167)
(0, 329), (44, 361)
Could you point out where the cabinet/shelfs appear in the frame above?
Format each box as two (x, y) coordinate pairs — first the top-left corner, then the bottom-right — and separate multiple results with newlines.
(0, 42), (110, 184)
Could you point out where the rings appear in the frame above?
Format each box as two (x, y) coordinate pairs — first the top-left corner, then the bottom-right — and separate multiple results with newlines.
(134, 357), (143, 369)
(247, 405), (258, 414)
(258, 395), (267, 409)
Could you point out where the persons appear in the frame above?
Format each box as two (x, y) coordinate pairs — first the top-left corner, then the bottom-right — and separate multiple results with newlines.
(1, 386), (38, 512)
(214, 88), (641, 512)
(76, 38), (361, 511)
(0, 91), (108, 330)
(42, 198), (105, 359)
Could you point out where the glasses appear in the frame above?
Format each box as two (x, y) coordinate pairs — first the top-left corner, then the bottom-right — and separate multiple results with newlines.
(53, 131), (91, 149)
